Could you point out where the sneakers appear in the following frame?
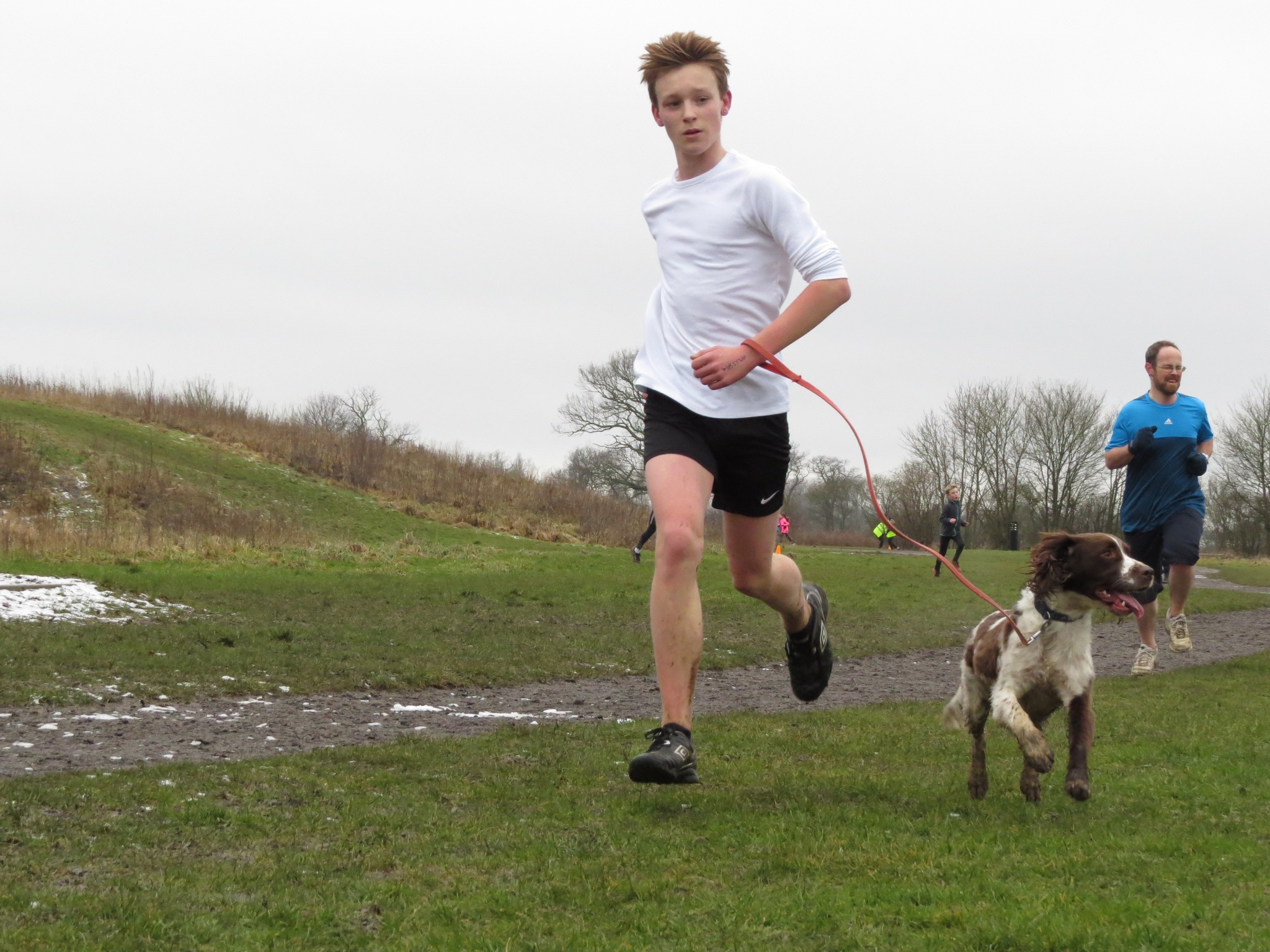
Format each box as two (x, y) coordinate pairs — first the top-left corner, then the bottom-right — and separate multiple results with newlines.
(932, 568), (939, 578)
(628, 727), (700, 784)
(952, 559), (960, 572)
(630, 547), (642, 563)
(1131, 643), (1159, 674)
(784, 582), (832, 701)
(1165, 607), (1192, 652)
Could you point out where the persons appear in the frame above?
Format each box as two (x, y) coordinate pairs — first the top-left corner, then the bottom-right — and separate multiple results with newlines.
(777, 513), (795, 546)
(933, 483), (970, 578)
(1162, 557), (1170, 583)
(873, 518), (900, 550)
(629, 32), (851, 787)
(1104, 341), (1215, 675)
(630, 511), (656, 563)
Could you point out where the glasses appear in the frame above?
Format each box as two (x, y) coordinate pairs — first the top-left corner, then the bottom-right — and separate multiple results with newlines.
(1154, 366), (1186, 373)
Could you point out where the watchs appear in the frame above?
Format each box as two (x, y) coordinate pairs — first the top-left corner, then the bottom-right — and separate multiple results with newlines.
(1200, 452), (1209, 461)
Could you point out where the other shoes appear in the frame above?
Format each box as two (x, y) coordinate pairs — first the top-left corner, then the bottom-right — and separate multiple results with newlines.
(1162, 574), (1168, 583)
(791, 543), (795, 545)
(896, 547), (900, 550)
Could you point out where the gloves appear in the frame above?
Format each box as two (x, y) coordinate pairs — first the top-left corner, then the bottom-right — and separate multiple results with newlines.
(1128, 426), (1158, 455)
(1188, 453), (1208, 477)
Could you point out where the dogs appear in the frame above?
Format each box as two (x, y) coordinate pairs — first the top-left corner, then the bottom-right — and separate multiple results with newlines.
(944, 528), (1154, 807)
